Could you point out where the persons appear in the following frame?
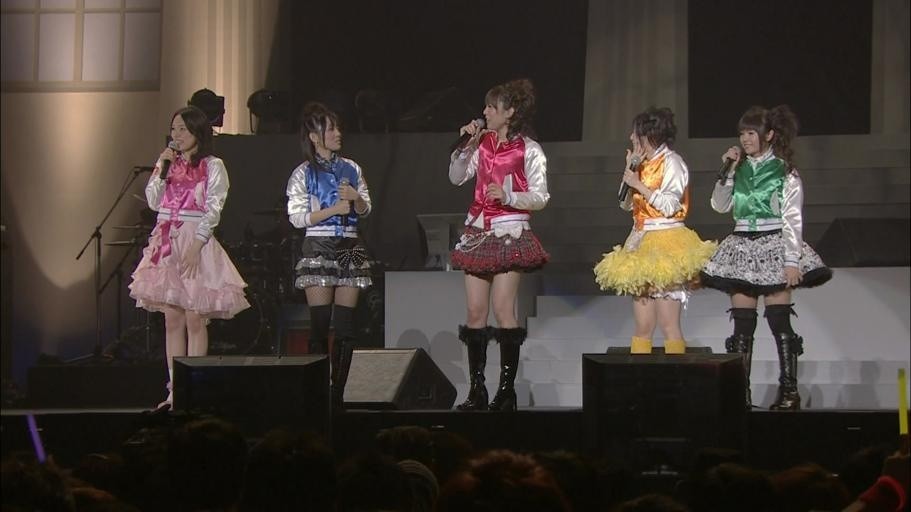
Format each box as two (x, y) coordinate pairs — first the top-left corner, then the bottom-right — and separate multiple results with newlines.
(618, 107), (692, 354)
(126, 106), (252, 410)
(0, 423), (911, 511)
(448, 79), (549, 413)
(287, 102), (372, 414)
(709, 105), (804, 409)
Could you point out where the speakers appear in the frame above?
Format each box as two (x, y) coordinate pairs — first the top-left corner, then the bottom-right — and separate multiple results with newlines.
(581, 352), (746, 450)
(172, 355), (332, 440)
(341, 346), (457, 411)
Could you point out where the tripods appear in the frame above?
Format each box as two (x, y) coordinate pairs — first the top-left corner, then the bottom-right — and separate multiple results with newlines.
(61, 171), (143, 364)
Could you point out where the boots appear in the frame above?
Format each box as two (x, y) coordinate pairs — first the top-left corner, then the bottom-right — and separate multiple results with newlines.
(455, 323), (489, 409)
(486, 327), (528, 411)
(768, 332), (804, 411)
(331, 334), (353, 409)
(724, 333), (754, 409)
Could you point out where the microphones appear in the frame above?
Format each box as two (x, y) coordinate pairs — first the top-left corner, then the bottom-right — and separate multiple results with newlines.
(618, 155), (641, 202)
(447, 118), (485, 153)
(134, 166), (155, 172)
(340, 176), (351, 232)
(160, 141), (178, 179)
(717, 145), (742, 181)
